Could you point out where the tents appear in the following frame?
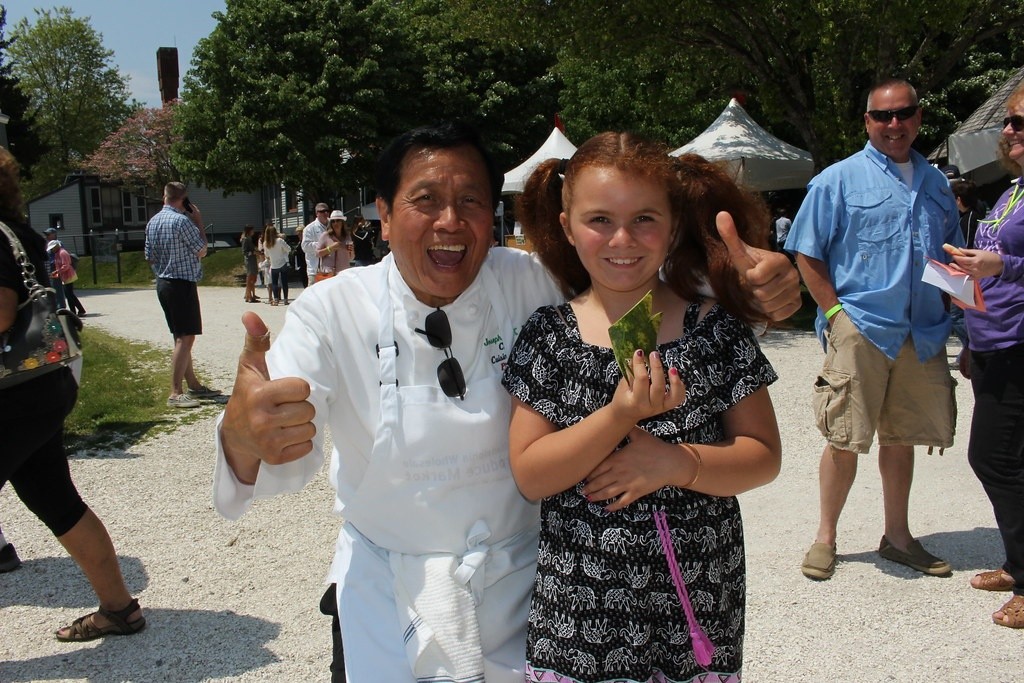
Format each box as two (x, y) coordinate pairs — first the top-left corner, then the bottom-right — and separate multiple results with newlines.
(667, 98), (815, 192)
(501, 126), (578, 247)
(948, 126), (1009, 187)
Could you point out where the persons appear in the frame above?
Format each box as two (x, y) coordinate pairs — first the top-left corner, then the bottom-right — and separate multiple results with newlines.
(775, 209), (792, 252)
(42, 228), (86, 317)
(784, 79), (967, 579)
(947, 180), (986, 368)
(0, 145), (146, 642)
(500, 132), (782, 683)
(214, 126), (802, 683)
(240, 203), (373, 306)
(949, 85), (1024, 627)
(144, 181), (222, 408)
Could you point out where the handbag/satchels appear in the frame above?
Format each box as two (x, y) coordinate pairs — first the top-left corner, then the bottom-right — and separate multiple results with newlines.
(0, 222), (83, 389)
(314, 272), (333, 283)
(68, 253), (80, 269)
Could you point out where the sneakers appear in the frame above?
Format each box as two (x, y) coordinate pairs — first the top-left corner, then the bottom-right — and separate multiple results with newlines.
(188, 386), (222, 398)
(168, 394), (200, 407)
(879, 535), (952, 575)
(800, 540), (837, 579)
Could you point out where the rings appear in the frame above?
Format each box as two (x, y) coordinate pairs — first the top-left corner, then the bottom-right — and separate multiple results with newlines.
(246, 330), (272, 353)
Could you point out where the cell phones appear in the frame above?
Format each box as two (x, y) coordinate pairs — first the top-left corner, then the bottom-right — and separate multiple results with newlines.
(182, 197), (193, 213)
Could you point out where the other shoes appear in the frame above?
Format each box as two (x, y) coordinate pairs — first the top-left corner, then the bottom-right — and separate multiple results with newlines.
(992, 596), (1024, 628)
(284, 299), (289, 305)
(970, 567), (1013, 590)
(244, 297), (261, 303)
(253, 295), (260, 299)
(0, 543), (20, 572)
(271, 298), (278, 306)
(265, 299), (273, 304)
(78, 311), (88, 317)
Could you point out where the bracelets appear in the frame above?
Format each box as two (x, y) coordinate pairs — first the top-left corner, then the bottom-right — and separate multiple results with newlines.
(678, 443), (702, 488)
(824, 304), (843, 320)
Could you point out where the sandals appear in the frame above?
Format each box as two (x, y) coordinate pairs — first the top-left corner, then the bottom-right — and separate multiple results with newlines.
(56, 598), (146, 641)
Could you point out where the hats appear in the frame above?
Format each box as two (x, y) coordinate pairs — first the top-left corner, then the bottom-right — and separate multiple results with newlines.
(41, 228), (57, 236)
(47, 240), (63, 252)
(940, 164), (960, 179)
(327, 210), (347, 222)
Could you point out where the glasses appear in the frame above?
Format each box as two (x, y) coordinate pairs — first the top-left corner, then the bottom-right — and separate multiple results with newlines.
(317, 209), (326, 213)
(331, 220), (341, 223)
(868, 105), (918, 122)
(1004, 115), (1024, 132)
(415, 306), (466, 401)
